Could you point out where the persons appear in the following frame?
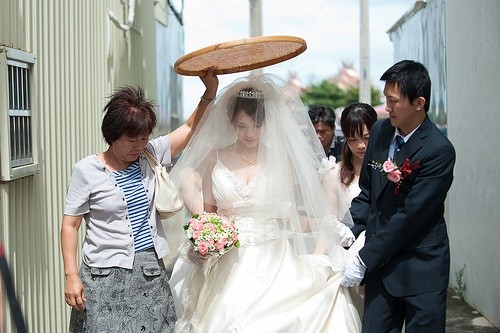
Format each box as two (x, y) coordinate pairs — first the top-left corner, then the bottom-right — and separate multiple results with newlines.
(61, 66), (218, 333)
(306, 106), (347, 164)
(161, 74), (365, 333)
(324, 103), (377, 222)
(340, 60), (456, 333)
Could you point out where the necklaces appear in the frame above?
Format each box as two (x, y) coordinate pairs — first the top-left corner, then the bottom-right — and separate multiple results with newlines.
(234, 143), (267, 165)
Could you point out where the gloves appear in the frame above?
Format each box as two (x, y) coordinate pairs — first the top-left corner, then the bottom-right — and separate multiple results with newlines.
(326, 218), (356, 247)
(338, 255), (366, 288)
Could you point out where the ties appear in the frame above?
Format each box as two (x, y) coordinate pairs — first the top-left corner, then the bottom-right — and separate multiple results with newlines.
(393, 135), (403, 168)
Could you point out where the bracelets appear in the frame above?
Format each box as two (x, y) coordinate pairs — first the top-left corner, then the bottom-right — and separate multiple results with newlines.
(201, 97), (215, 102)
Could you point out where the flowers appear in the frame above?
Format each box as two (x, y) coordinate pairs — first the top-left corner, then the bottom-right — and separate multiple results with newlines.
(183, 210), (240, 256)
(367, 156), (403, 187)
